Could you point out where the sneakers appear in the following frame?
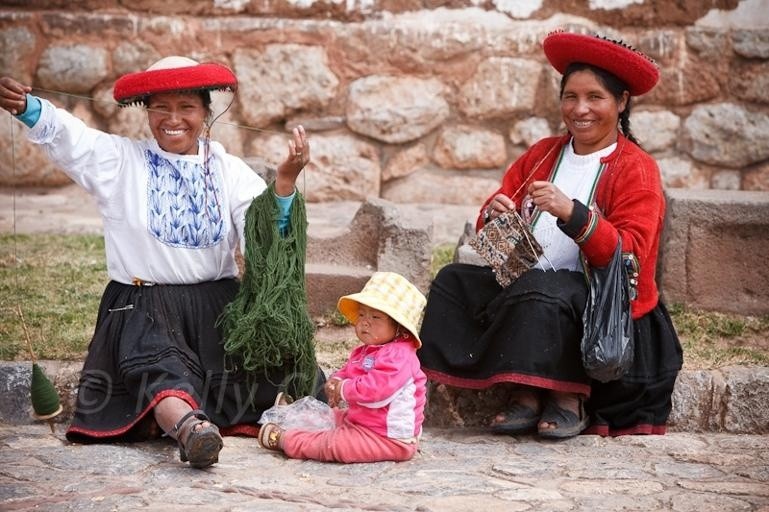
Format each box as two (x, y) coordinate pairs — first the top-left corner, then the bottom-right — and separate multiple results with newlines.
(258, 421), (289, 453)
(275, 391), (294, 408)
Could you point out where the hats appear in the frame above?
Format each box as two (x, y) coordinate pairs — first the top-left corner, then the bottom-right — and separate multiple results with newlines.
(112, 53), (238, 107)
(542, 29), (660, 98)
(337, 270), (427, 350)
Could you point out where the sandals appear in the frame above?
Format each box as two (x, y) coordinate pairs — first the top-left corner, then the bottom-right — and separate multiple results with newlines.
(492, 388), (541, 436)
(537, 401), (596, 436)
(161, 408), (223, 469)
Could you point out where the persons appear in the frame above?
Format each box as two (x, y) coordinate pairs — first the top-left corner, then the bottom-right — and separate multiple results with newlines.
(254, 271), (430, 467)
(417, 26), (686, 442)
(0, 54), (312, 469)
